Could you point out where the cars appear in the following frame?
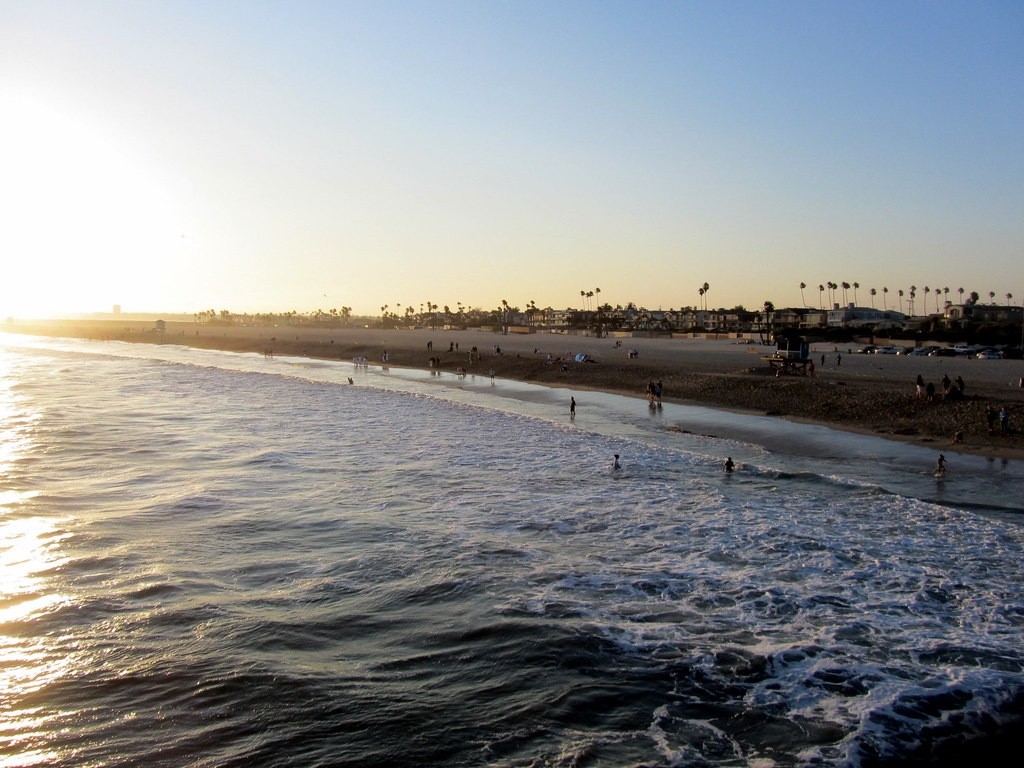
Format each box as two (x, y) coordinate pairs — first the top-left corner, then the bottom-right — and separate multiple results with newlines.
(857, 344), (1005, 359)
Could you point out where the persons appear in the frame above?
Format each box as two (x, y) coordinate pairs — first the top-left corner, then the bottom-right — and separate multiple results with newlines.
(568, 396), (577, 416)
(612, 453), (621, 470)
(936, 454), (950, 481)
(721, 456), (734, 474)
(78, 329), (1010, 445)
